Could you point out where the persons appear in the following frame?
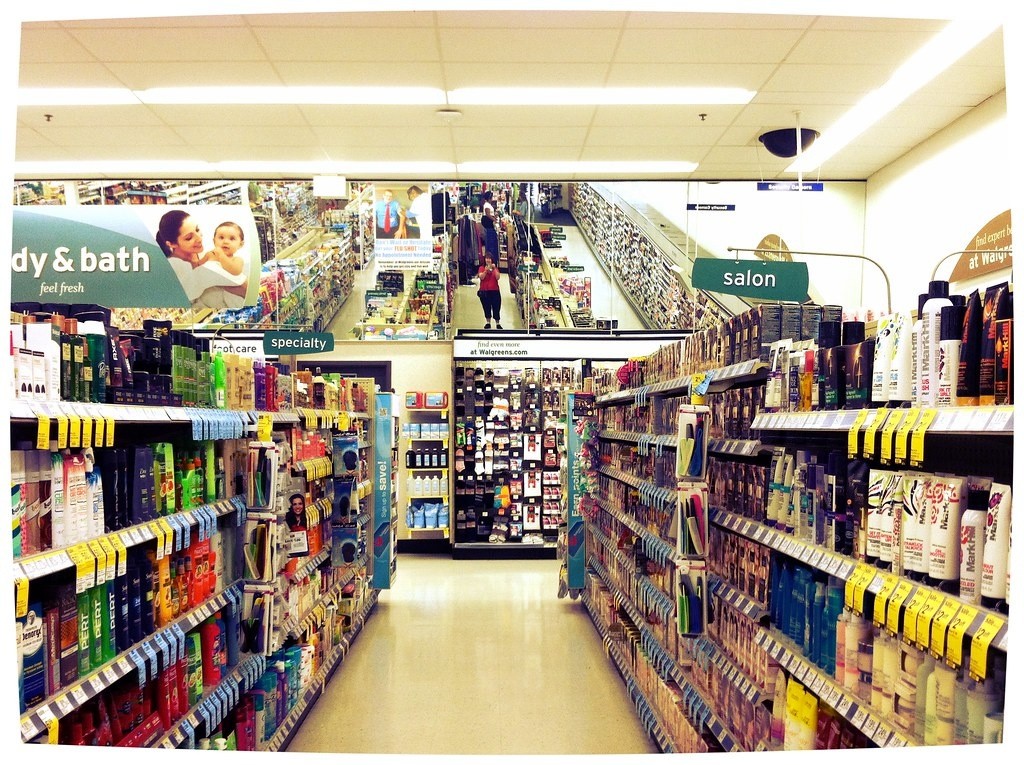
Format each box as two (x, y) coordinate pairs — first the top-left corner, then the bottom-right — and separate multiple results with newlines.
(375, 187), (405, 238)
(399, 184), (429, 239)
(512, 189), (528, 222)
(284, 493), (307, 531)
(476, 251), (504, 329)
(199, 219), (248, 308)
(155, 209), (246, 307)
(480, 190), (499, 252)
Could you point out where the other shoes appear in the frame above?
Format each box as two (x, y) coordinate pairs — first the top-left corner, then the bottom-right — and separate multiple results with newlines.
(484, 324), (491, 328)
(497, 324), (503, 329)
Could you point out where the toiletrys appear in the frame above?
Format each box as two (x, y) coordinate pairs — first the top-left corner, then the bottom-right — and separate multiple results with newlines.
(558, 278), (1015, 751)
(348, 233), (456, 339)
(507, 223), (568, 329)
(9, 298), (397, 751)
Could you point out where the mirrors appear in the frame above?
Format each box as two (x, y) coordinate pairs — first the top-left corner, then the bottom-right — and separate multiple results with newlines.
(12, 177), (867, 345)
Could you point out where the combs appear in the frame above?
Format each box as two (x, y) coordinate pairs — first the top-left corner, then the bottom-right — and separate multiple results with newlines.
(674, 403), (711, 636)
(240, 441), (277, 653)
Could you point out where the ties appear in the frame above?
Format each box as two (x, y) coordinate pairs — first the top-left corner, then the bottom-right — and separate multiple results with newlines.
(384, 204), (391, 233)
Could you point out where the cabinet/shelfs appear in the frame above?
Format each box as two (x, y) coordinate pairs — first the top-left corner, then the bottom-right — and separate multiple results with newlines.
(569, 182), (781, 333)
(10, 395), (403, 752)
(397, 393), (452, 554)
(560, 357), (1013, 754)
(445, 337), (632, 561)
(17, 180), (376, 333)
(345, 266), (453, 339)
